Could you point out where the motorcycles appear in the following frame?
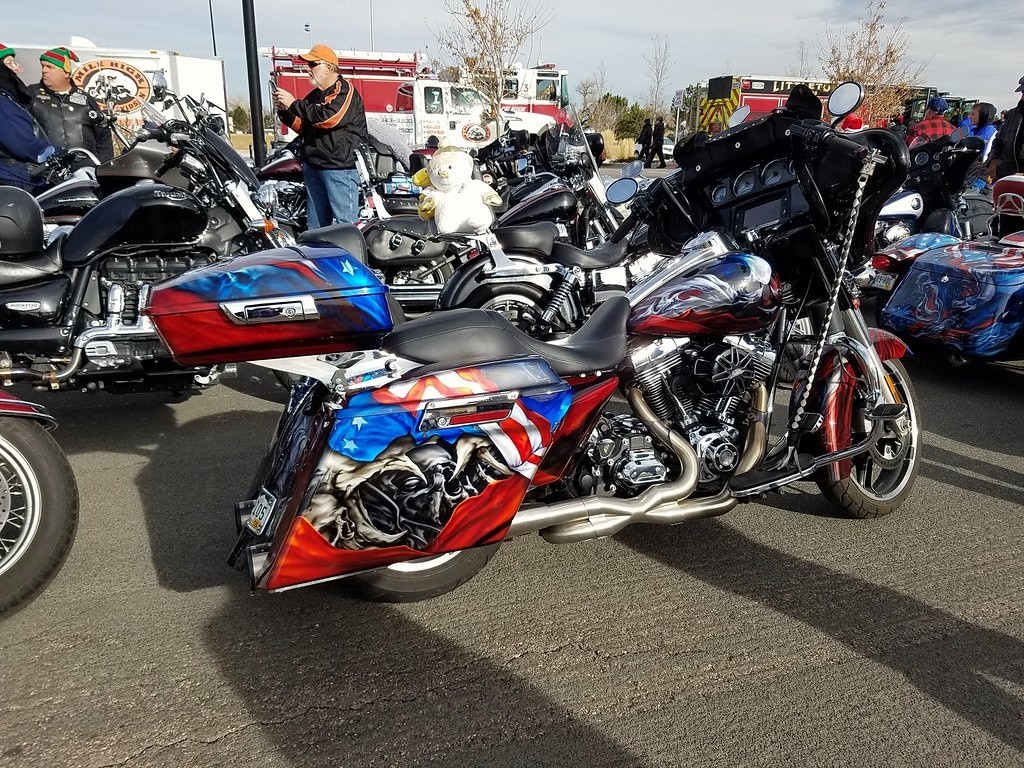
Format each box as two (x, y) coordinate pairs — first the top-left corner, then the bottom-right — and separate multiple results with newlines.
(218, 82), (924, 607)
(1, 71), (1023, 623)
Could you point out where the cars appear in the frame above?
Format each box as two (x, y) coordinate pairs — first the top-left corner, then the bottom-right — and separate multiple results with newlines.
(633, 135), (675, 159)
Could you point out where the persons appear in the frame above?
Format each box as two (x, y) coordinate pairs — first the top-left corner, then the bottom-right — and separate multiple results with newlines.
(271, 45), (368, 230)
(0, 43), (114, 199)
(831, 77), (1024, 184)
(634, 117), (688, 169)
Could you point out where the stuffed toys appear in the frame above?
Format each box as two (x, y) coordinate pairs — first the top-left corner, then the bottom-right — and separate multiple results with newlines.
(413, 145), (503, 237)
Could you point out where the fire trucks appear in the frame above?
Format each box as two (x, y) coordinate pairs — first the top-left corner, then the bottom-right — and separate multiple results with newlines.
(697, 73), (980, 141)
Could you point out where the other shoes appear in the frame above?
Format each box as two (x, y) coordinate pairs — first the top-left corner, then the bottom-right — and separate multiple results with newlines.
(676, 165), (680, 168)
(644, 165), (650, 168)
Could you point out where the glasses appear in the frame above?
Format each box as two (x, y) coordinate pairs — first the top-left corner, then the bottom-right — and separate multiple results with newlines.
(308, 61), (327, 68)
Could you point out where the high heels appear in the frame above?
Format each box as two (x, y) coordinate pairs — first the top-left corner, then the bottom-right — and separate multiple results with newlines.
(658, 161), (666, 168)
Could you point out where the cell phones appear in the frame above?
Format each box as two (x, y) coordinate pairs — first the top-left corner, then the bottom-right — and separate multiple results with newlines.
(268, 80), (277, 92)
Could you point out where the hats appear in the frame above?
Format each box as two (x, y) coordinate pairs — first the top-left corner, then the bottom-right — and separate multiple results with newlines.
(680, 121), (687, 128)
(657, 117), (663, 123)
(39, 47), (79, 75)
(0, 43), (15, 59)
(924, 98), (948, 113)
(298, 45), (338, 66)
(644, 118), (650, 124)
(1014, 76), (1024, 92)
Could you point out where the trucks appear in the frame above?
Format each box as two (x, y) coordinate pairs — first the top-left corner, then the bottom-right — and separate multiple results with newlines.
(258, 46), (575, 157)
